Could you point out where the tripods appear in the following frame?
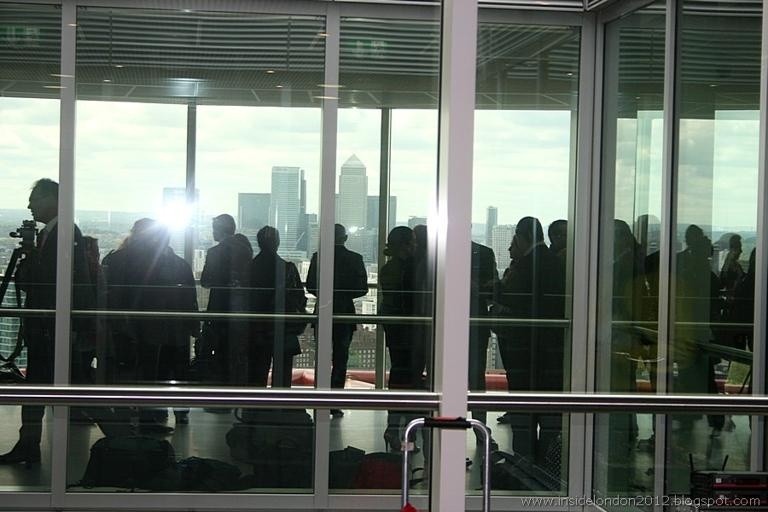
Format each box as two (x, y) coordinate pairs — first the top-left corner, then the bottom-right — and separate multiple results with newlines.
(0, 244), (48, 380)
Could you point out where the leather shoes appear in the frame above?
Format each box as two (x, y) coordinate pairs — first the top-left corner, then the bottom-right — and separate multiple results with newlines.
(1, 438), (41, 465)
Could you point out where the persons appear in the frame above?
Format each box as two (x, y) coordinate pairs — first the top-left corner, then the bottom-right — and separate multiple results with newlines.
(0, 173), (92, 470)
(74, 214), (757, 470)
